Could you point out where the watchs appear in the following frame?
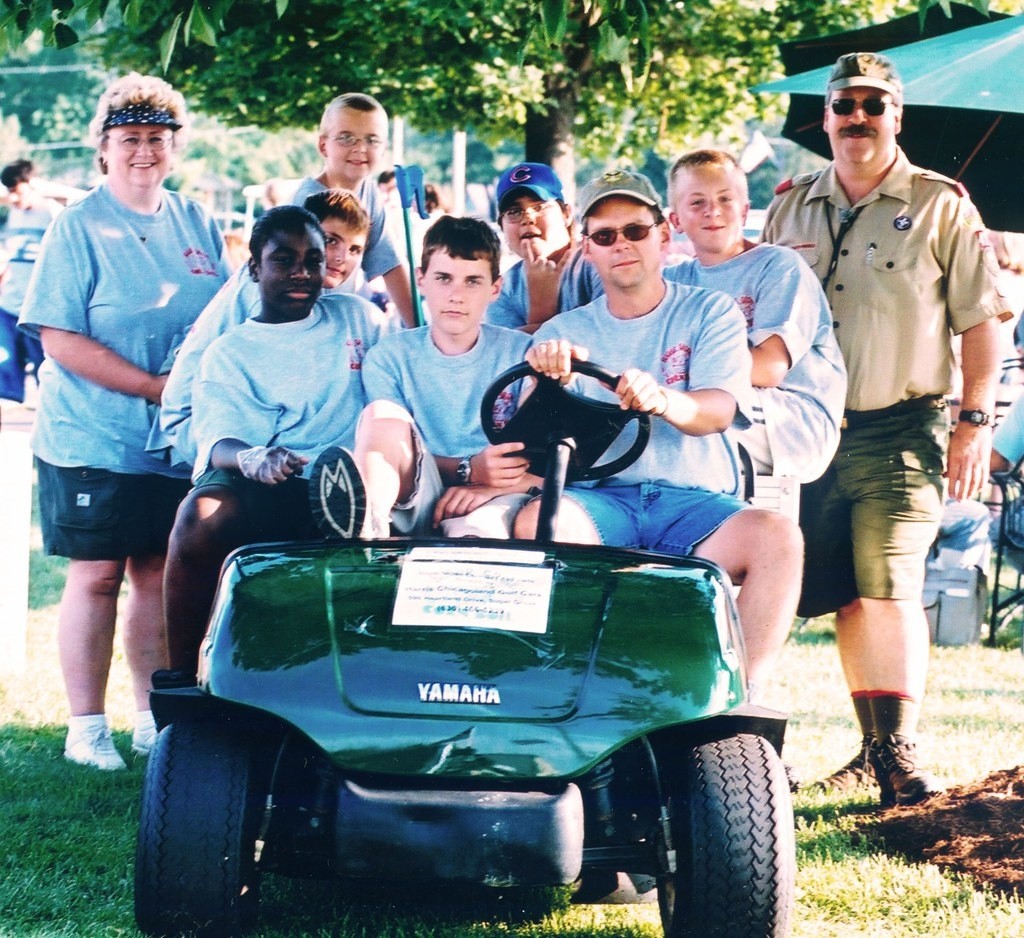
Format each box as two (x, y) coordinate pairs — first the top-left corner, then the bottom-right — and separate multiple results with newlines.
(956, 409), (994, 426)
(456, 455), (476, 485)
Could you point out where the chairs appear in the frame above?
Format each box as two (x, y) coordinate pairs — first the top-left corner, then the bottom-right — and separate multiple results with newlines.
(989, 455), (1024, 647)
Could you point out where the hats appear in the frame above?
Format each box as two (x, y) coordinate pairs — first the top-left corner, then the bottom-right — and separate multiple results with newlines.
(829, 52), (902, 96)
(578, 169), (662, 225)
(496, 162), (564, 212)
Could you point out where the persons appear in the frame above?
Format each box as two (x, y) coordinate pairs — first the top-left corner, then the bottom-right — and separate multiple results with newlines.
(0, 159), (64, 426)
(916, 229), (1024, 573)
(761, 52), (1015, 806)
(154, 93), (851, 678)
(14, 74), (236, 772)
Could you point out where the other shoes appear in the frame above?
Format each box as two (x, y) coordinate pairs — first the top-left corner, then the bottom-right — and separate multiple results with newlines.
(308, 447), (377, 540)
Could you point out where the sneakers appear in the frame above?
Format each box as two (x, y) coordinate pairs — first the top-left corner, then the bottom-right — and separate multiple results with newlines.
(874, 733), (928, 806)
(131, 719), (159, 755)
(815, 734), (879, 796)
(64, 725), (128, 770)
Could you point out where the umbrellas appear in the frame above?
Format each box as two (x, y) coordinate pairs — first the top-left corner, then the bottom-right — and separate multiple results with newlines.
(749, 0), (1024, 233)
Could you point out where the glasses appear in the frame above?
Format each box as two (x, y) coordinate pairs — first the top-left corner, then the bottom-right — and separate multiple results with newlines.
(105, 132), (173, 151)
(827, 97), (897, 116)
(499, 204), (560, 224)
(325, 134), (384, 148)
(585, 219), (665, 246)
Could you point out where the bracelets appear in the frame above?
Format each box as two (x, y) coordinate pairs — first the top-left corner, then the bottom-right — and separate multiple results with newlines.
(657, 386), (670, 415)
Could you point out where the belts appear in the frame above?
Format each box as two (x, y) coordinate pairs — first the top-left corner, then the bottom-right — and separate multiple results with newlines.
(840, 393), (946, 430)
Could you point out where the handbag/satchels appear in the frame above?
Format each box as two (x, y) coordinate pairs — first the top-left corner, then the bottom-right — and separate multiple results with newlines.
(921, 564), (989, 646)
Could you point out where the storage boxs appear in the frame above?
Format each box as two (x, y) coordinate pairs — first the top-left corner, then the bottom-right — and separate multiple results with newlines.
(923, 564), (988, 645)
